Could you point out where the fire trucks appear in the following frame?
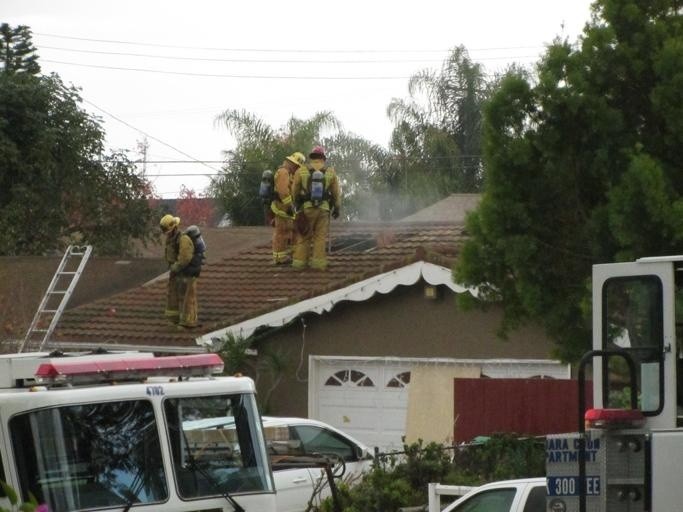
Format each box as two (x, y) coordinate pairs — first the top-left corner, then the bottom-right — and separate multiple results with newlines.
(0, 244), (279, 512)
(546, 255), (683, 512)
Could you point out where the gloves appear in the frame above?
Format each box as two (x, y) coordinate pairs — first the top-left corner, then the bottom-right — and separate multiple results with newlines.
(286, 204), (296, 217)
(332, 208), (341, 220)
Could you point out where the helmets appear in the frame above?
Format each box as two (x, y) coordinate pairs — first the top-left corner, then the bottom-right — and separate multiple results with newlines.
(309, 146), (327, 160)
(160, 214), (181, 235)
(286, 151), (306, 168)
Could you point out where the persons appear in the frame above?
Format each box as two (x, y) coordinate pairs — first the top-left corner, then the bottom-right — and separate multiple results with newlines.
(270, 146), (342, 272)
(159, 214), (200, 329)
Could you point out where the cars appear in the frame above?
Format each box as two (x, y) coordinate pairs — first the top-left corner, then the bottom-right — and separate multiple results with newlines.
(182, 414), (397, 512)
(441, 477), (547, 512)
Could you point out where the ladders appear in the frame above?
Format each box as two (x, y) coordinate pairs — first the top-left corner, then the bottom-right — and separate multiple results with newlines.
(17, 243), (92, 353)
